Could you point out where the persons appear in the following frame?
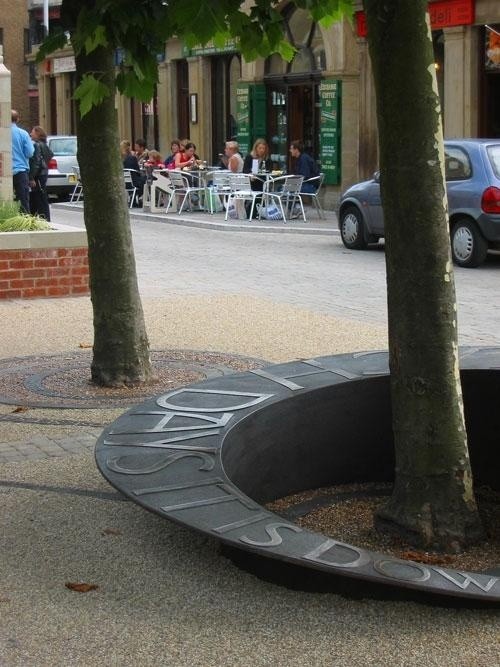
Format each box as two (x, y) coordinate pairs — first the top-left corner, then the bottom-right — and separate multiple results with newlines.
(11, 107), (38, 212)
(26, 124), (55, 223)
(118, 135), (321, 222)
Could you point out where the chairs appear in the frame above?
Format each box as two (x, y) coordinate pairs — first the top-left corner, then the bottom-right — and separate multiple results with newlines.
(68, 156), (326, 224)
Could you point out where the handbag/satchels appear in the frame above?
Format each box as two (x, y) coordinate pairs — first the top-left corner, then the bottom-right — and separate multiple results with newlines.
(38, 142), (47, 170)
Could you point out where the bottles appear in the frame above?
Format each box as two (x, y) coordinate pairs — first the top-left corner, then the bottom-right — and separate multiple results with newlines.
(261, 160), (267, 175)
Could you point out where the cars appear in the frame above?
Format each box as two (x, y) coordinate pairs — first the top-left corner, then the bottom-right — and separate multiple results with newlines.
(46, 135), (82, 199)
(335, 137), (500, 267)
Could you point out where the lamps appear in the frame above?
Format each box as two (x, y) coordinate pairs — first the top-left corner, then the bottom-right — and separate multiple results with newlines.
(189, 90), (197, 125)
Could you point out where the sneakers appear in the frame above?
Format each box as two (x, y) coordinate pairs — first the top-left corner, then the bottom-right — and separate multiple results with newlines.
(290, 206), (307, 219)
